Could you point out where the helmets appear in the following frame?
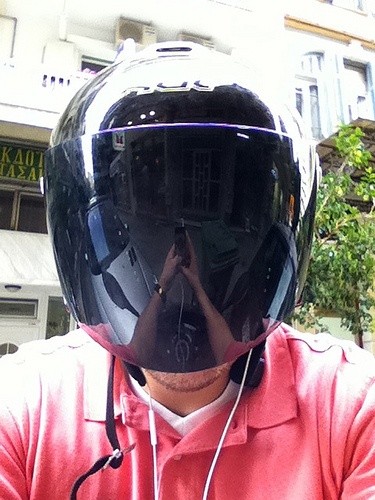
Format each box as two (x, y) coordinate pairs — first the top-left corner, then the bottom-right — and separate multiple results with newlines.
(38, 40), (322, 374)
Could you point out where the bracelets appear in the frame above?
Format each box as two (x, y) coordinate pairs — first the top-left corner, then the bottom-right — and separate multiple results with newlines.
(154, 284), (166, 303)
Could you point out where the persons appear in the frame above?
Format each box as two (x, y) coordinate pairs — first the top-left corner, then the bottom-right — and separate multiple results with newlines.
(0, 40), (374, 500)
(123, 218), (244, 367)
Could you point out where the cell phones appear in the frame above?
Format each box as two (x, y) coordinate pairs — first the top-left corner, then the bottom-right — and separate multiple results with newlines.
(174, 226), (190, 264)
